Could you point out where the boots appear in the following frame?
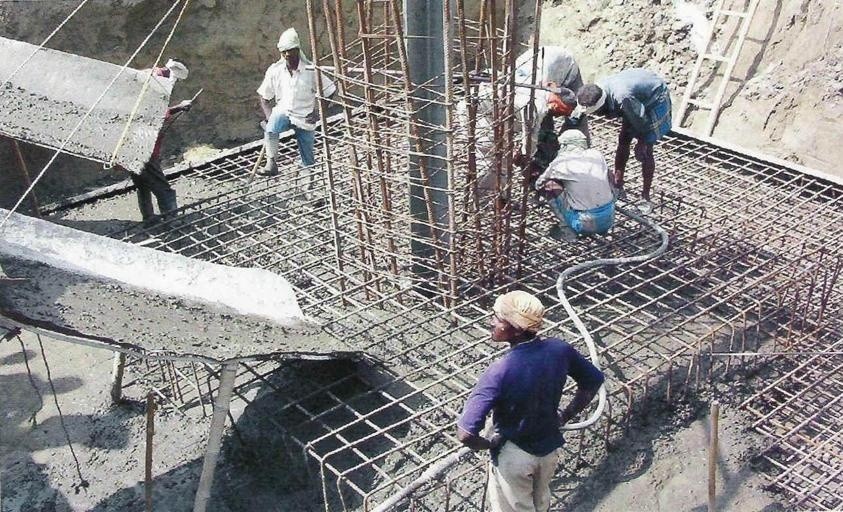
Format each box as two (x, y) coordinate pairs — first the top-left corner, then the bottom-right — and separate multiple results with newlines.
(300, 165), (317, 200)
(255, 131), (280, 177)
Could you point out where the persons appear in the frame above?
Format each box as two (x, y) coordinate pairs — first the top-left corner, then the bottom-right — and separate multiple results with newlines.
(580, 68), (673, 200)
(535, 130), (615, 243)
(121, 58), (193, 225)
(453, 289), (606, 510)
(455, 44), (588, 215)
(252, 27), (337, 208)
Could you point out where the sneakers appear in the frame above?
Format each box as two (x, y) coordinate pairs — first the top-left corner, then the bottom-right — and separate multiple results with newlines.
(639, 198), (655, 218)
